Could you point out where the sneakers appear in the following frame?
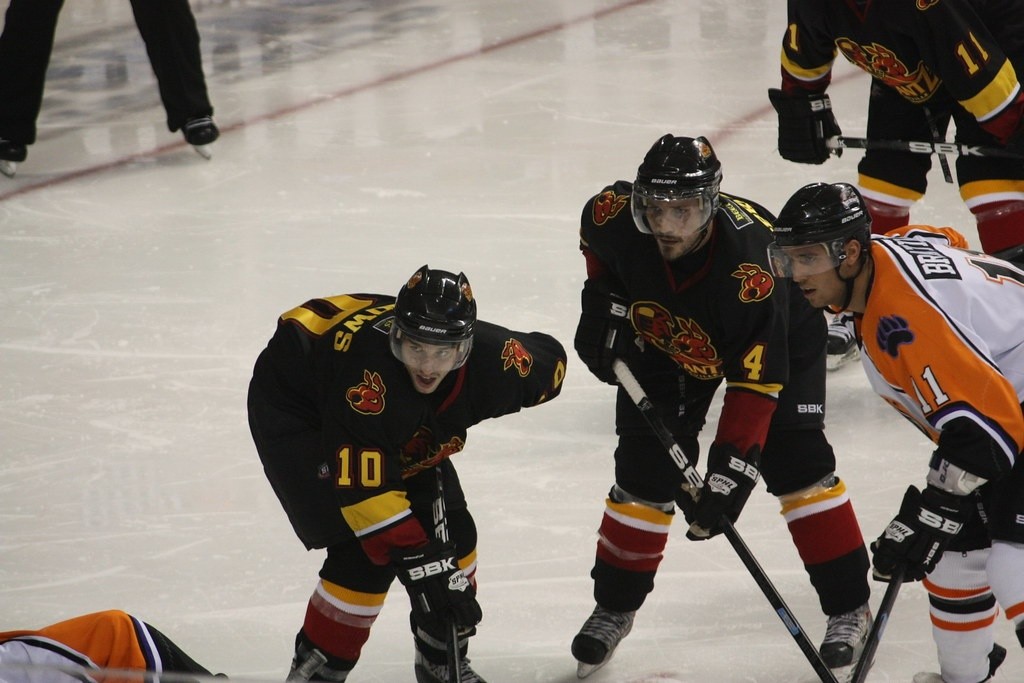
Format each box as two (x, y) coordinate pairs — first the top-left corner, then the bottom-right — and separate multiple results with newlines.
(0, 139), (27, 175)
(414, 645), (489, 683)
(819, 601), (876, 681)
(825, 312), (862, 372)
(913, 672), (944, 683)
(182, 107), (220, 159)
(571, 600), (635, 678)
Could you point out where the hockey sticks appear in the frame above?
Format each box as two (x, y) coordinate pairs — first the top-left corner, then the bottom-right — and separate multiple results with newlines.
(428, 467), (461, 682)
(849, 576), (906, 683)
(828, 135), (1023, 160)
(612, 358), (838, 683)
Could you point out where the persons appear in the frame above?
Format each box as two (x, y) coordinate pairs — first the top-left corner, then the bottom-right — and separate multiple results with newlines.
(0, 609), (231, 683)
(0, 0), (219, 177)
(572, 133), (874, 683)
(247, 264), (567, 683)
(767, 183), (1024, 683)
(768, 0), (1024, 371)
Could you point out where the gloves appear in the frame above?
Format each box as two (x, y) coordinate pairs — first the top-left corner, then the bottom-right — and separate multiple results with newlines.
(673, 440), (766, 541)
(870, 485), (969, 583)
(768, 88), (843, 166)
(574, 278), (641, 385)
(391, 539), (483, 628)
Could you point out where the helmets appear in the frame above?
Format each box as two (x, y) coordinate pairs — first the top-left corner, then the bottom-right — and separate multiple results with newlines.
(634, 133), (723, 200)
(393, 264), (477, 346)
(772, 182), (872, 246)
